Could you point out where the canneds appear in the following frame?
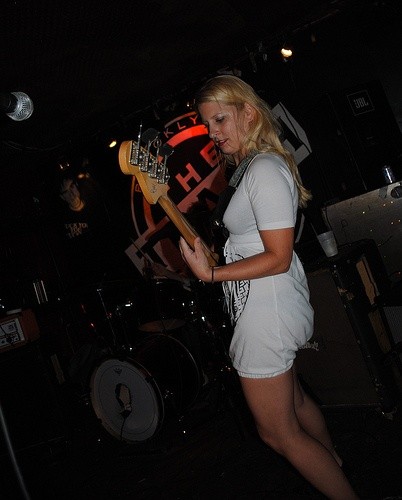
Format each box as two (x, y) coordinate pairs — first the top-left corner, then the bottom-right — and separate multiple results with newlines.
(382, 165), (395, 185)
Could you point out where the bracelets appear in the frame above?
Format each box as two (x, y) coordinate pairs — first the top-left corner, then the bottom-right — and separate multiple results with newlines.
(212, 266), (213, 281)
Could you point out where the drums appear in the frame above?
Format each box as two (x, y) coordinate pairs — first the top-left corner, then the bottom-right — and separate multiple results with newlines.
(88, 333), (204, 443)
(125, 281), (189, 334)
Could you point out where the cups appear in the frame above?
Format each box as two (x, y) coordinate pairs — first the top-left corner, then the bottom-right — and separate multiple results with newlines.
(316, 230), (338, 258)
(32, 279), (48, 304)
(382, 166), (396, 183)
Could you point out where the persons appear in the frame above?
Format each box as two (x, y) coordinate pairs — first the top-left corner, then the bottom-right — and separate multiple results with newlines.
(178, 75), (359, 500)
(52, 176), (96, 237)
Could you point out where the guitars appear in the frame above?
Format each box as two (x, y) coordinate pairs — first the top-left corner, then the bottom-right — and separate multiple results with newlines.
(118, 134), (220, 268)
